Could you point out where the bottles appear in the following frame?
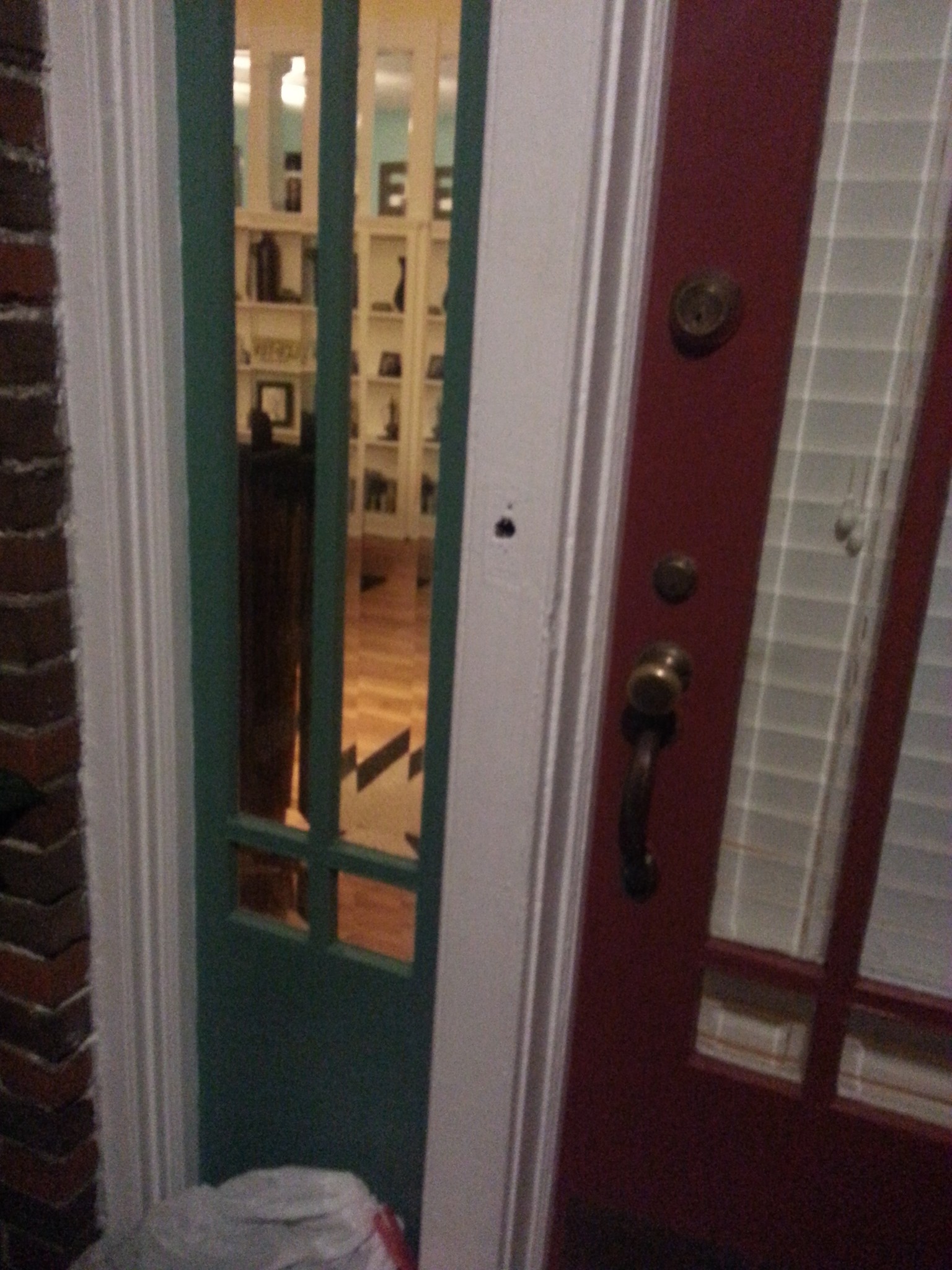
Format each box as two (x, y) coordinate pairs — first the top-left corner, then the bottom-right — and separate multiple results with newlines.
(256, 231), (281, 302)
(393, 255), (405, 313)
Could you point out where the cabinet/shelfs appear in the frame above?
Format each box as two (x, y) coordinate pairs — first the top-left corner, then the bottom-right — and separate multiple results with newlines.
(223, 209), (455, 625)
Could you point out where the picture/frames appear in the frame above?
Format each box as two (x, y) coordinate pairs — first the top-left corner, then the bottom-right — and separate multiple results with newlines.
(254, 380), (295, 427)
